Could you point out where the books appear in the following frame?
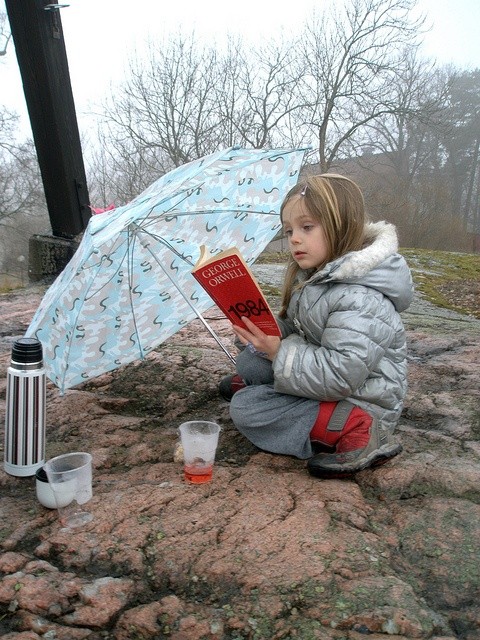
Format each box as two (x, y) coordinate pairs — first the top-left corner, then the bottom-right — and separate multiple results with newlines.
(190, 244), (283, 338)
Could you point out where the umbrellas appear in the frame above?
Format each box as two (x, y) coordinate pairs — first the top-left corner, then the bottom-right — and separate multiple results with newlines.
(24, 144), (313, 398)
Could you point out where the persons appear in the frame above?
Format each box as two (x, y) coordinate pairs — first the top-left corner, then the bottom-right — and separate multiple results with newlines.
(219, 173), (413, 479)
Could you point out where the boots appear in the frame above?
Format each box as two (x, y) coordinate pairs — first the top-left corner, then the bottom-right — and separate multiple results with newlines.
(220, 374), (245, 401)
(307, 400), (403, 478)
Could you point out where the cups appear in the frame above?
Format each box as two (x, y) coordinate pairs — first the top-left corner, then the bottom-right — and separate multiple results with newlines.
(42, 451), (98, 525)
(178, 419), (223, 485)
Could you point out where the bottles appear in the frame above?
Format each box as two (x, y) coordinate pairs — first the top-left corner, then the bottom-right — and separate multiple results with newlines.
(4, 337), (46, 479)
